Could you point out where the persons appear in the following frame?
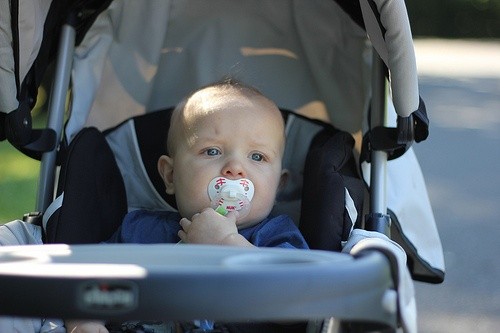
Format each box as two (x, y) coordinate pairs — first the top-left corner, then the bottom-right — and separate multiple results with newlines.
(58, 83), (309, 333)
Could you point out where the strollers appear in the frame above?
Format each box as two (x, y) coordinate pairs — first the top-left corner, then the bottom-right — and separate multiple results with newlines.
(1, 0), (449, 333)
